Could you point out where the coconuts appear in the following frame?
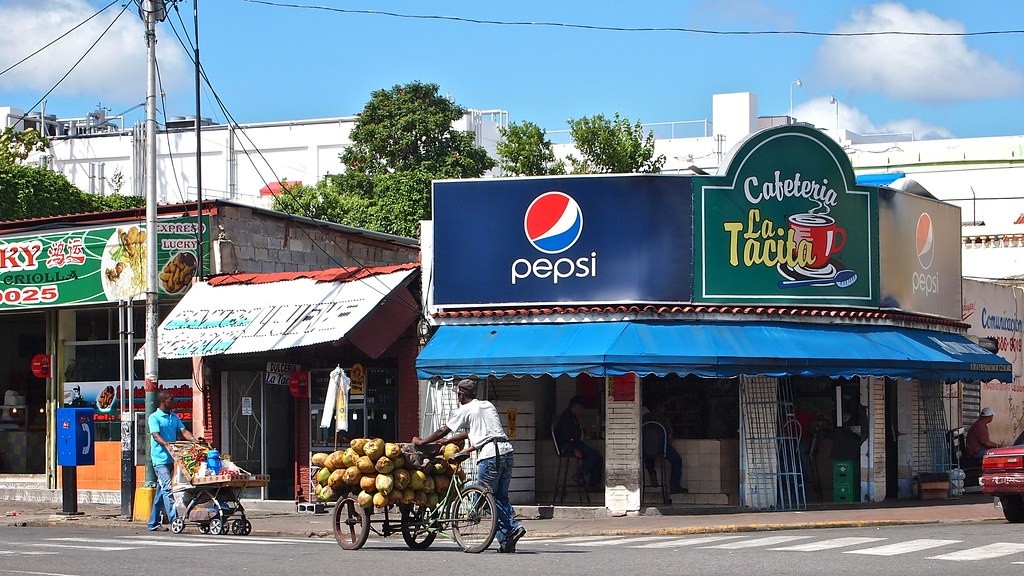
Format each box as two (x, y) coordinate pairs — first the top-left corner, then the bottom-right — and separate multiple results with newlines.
(311, 438), (460, 507)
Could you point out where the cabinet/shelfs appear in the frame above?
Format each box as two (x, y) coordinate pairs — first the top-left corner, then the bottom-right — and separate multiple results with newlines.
(307, 365), (398, 507)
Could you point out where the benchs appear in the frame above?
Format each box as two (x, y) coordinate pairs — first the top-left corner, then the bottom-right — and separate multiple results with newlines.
(948, 427), (983, 487)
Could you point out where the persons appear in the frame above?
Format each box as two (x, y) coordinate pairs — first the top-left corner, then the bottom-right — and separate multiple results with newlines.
(148, 392), (206, 530)
(966, 408), (1009, 485)
(553, 395), (604, 492)
(642, 396), (688, 494)
(68, 386), (85, 407)
(779, 398), (818, 503)
(412, 379), (526, 553)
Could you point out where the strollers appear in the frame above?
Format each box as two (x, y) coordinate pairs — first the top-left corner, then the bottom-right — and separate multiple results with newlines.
(166, 437), (253, 537)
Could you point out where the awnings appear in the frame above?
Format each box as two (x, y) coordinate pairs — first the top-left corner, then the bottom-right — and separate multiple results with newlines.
(134, 269), (419, 360)
(415, 320), (1012, 383)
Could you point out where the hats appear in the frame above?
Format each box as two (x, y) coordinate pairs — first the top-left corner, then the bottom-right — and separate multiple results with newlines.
(570, 395), (590, 408)
(452, 379), (478, 397)
(979, 407), (997, 416)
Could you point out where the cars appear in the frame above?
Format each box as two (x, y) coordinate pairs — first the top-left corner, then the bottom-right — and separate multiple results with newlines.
(978, 427), (1024, 524)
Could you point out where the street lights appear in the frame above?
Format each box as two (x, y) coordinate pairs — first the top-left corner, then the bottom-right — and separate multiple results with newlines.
(829, 94), (839, 144)
(790, 78), (803, 126)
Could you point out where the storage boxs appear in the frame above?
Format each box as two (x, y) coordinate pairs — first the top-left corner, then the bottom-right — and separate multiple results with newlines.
(833, 460), (854, 503)
(921, 481), (950, 499)
(166, 440), (270, 487)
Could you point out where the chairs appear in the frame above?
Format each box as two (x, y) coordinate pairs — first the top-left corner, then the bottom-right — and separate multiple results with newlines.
(641, 421), (672, 505)
(551, 423), (591, 504)
(778, 418), (825, 501)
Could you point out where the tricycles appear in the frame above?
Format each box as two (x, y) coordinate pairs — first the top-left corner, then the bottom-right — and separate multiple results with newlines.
(331, 439), (498, 554)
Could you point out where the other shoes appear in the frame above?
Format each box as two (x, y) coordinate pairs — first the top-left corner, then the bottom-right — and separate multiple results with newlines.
(504, 527), (526, 553)
(651, 480), (658, 487)
(154, 525), (170, 532)
(670, 486), (689, 494)
(496, 545), (515, 553)
(571, 473), (585, 485)
